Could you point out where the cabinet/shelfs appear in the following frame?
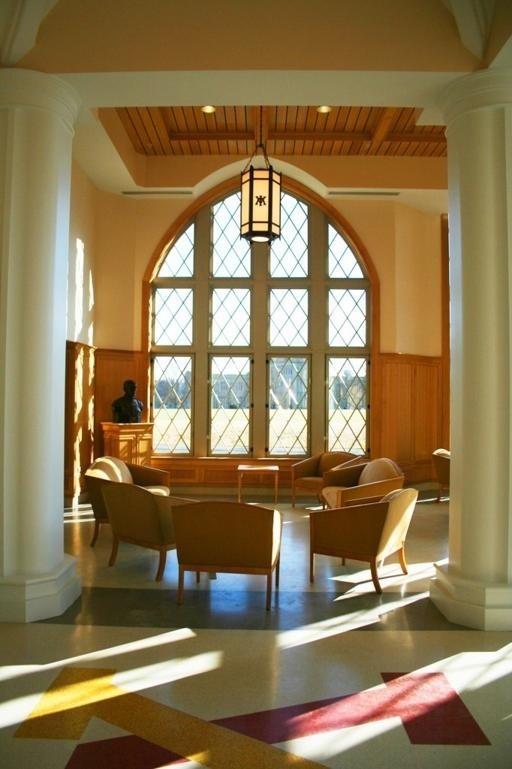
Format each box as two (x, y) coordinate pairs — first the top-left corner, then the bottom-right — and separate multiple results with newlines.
(100, 421), (155, 468)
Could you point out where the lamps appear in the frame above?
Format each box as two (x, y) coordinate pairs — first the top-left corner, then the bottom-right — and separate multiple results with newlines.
(238, 106), (282, 247)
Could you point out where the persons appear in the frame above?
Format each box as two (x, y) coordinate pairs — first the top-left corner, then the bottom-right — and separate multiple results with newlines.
(112, 379), (144, 423)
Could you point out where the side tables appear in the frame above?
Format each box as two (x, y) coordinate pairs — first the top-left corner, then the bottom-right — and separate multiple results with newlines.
(237, 464), (279, 504)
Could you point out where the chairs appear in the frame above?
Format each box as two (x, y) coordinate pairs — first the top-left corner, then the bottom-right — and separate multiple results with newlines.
(102, 482), (202, 582)
(321, 457), (405, 510)
(290, 451), (361, 508)
(84, 455), (170, 548)
(170, 500), (283, 611)
(431, 448), (451, 503)
(308, 487), (420, 596)
